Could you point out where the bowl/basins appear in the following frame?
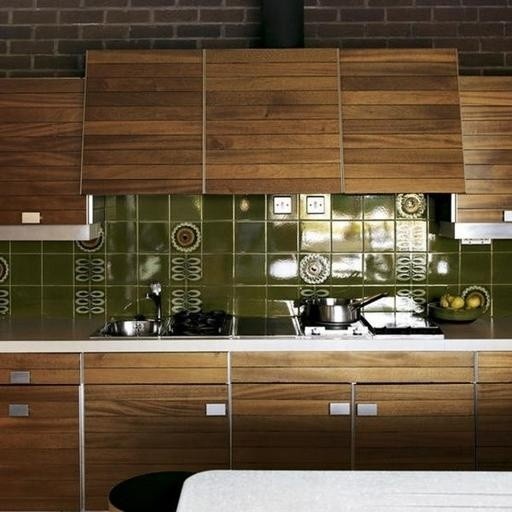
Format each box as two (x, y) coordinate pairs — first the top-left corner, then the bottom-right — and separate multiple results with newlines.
(111, 320), (158, 336)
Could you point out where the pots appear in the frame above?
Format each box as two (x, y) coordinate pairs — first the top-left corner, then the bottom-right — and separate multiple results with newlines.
(297, 287), (388, 323)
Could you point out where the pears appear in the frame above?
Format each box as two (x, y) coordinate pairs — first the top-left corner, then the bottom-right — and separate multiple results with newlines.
(440, 291), (481, 309)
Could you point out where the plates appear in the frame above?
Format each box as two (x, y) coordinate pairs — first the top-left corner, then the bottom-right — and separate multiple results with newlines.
(430, 302), (483, 323)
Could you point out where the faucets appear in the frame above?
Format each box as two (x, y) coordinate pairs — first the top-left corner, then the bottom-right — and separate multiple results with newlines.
(145, 281), (162, 320)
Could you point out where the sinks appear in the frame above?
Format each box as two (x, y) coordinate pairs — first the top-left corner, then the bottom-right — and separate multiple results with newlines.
(88, 317), (163, 338)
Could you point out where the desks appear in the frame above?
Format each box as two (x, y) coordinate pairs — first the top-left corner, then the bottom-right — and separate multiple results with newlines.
(173, 468), (512, 512)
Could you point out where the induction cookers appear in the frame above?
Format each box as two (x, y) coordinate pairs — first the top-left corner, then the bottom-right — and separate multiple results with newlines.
(293, 311), (445, 340)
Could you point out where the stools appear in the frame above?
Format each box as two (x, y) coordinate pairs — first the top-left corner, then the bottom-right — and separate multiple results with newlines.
(105, 471), (197, 511)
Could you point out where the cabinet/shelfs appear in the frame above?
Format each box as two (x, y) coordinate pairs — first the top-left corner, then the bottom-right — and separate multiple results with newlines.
(232, 351), (476, 470)
(0, 353), (230, 512)
(84, 46), (204, 194)
(343, 46), (465, 195)
(474, 351), (512, 471)
(438, 74), (512, 240)
(0, 77), (109, 241)
(205, 46), (340, 195)
